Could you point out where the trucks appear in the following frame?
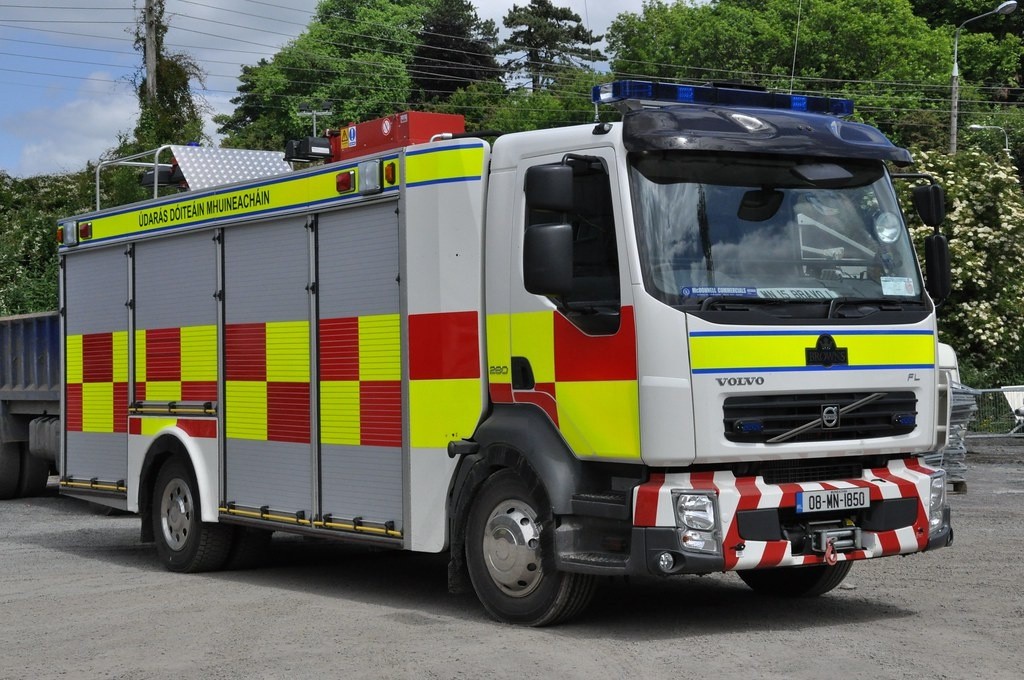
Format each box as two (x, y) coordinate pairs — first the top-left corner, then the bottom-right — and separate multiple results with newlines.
(0, 311), (60, 499)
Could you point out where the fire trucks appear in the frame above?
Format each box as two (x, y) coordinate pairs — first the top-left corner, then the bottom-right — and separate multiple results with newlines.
(56, 81), (954, 627)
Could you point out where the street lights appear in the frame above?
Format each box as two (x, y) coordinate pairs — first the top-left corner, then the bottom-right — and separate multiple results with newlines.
(967, 125), (1009, 150)
(296, 101), (332, 138)
(952, 0), (1017, 155)
